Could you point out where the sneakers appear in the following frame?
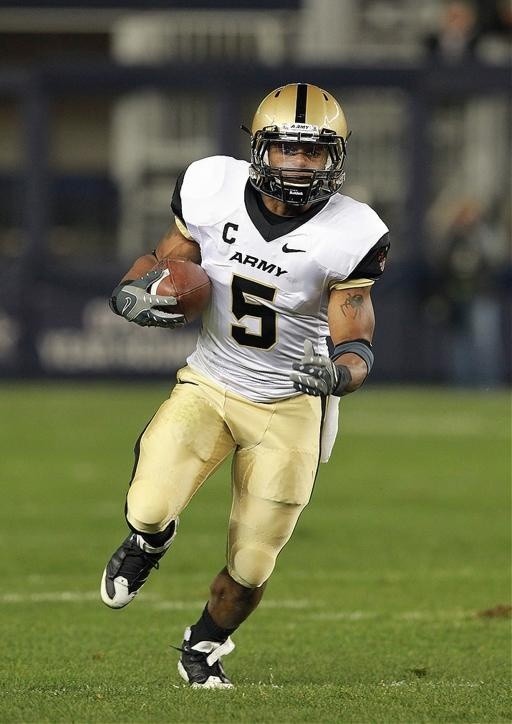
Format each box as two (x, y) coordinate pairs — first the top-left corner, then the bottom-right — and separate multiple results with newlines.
(100, 516), (180, 610)
(169, 626), (236, 689)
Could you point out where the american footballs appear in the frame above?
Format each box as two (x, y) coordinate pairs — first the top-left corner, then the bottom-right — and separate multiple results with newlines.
(146, 256), (211, 326)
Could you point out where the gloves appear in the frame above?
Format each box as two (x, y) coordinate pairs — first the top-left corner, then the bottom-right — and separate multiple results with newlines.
(289, 339), (353, 396)
(108, 269), (187, 329)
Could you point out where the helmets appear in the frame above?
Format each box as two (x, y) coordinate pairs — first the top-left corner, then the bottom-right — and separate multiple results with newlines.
(248, 81), (349, 184)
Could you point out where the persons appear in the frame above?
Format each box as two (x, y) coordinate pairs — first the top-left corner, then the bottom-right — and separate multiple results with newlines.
(100, 83), (391, 690)
